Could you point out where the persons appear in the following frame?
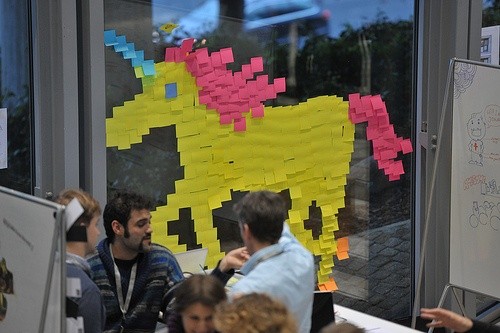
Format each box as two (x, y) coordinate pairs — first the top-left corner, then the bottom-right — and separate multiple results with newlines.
(166, 274), (228, 333)
(86, 192), (251, 333)
(419, 306), (500, 333)
(223, 189), (314, 333)
(212, 293), (366, 333)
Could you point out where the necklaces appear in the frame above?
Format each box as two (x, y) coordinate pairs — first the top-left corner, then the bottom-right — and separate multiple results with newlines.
(49, 188), (107, 333)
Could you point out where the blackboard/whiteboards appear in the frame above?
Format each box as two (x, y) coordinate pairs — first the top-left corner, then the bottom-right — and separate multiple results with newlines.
(447, 56), (500, 298)
(0, 187), (65, 331)
